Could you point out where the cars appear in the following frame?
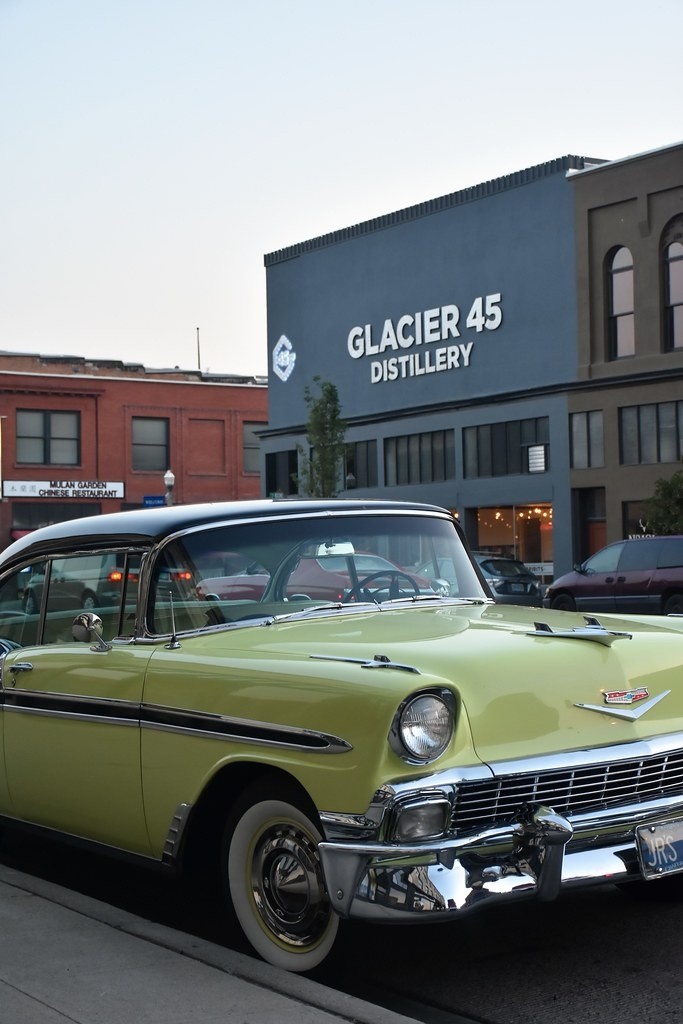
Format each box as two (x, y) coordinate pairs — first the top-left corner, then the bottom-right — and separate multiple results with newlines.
(19, 549), (193, 616)
(193, 546), (452, 608)
(410, 552), (544, 610)
(0, 500), (683, 980)
(543, 534), (683, 618)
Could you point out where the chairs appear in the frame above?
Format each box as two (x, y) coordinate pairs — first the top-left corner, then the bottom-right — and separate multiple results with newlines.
(62, 611), (210, 643)
(207, 600), (333, 624)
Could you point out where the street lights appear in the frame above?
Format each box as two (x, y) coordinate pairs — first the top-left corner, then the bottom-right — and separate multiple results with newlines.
(162, 469), (175, 507)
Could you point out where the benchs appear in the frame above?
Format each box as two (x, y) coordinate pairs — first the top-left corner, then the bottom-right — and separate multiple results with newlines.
(0, 601), (257, 648)
